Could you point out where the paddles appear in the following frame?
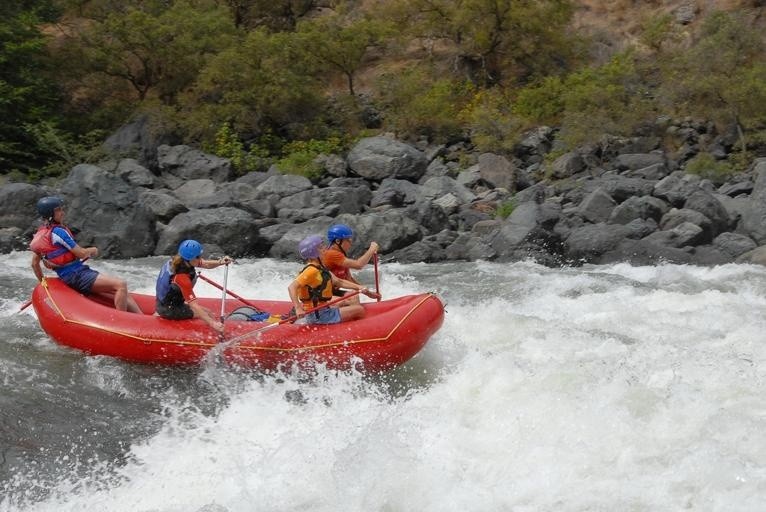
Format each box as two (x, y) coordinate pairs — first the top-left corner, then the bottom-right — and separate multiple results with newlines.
(225, 291), (362, 348)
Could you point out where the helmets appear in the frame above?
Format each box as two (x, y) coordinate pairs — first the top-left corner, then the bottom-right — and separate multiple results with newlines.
(37, 196), (64, 219)
(178, 240), (203, 261)
(298, 235), (325, 258)
(327, 224), (353, 242)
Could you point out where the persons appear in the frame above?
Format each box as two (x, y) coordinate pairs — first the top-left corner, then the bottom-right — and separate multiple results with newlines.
(288, 224), (381, 325)
(31, 197), (143, 314)
(156, 239), (233, 333)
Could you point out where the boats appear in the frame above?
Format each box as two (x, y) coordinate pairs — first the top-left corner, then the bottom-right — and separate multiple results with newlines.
(31, 273), (447, 375)
